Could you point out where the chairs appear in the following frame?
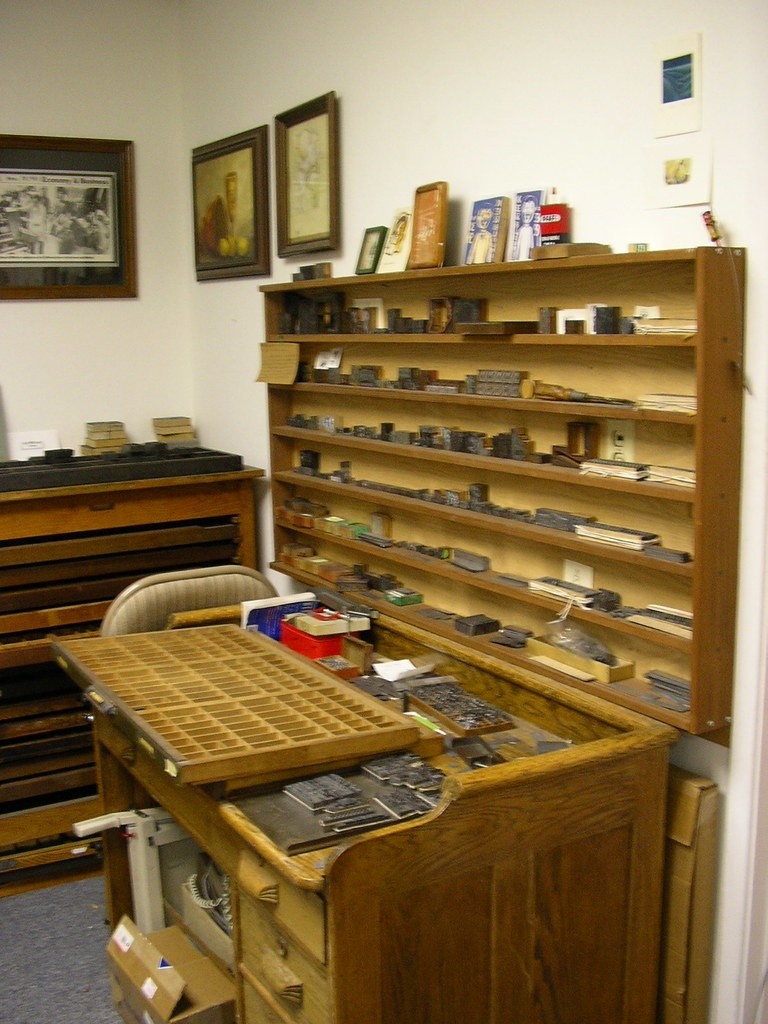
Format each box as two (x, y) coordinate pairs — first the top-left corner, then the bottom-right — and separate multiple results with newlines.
(101, 564), (279, 638)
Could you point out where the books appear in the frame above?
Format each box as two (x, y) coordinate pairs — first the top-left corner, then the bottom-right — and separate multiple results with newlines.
(625, 602), (693, 640)
(529, 575), (600, 609)
(463, 195), (511, 263)
(507, 188), (549, 260)
(539, 202), (569, 246)
(643, 463), (697, 489)
(572, 520), (661, 551)
(634, 393), (698, 418)
(530, 241), (611, 259)
(578, 456), (651, 482)
(633, 315), (698, 335)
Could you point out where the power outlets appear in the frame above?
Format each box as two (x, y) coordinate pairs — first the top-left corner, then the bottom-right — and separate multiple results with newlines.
(607, 419), (635, 463)
(562, 559), (594, 588)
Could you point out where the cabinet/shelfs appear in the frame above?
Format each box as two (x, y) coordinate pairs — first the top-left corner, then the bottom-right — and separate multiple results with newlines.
(259, 244), (749, 748)
(82, 689), (681, 1024)
(1, 465), (266, 894)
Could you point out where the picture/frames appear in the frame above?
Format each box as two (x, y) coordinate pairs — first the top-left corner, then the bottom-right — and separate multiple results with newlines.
(528, 242), (611, 259)
(355, 226), (388, 275)
(642, 144), (712, 210)
(0, 134), (138, 300)
(653, 32), (703, 140)
(274, 89), (340, 259)
(192, 124), (272, 282)
(410, 181), (448, 269)
